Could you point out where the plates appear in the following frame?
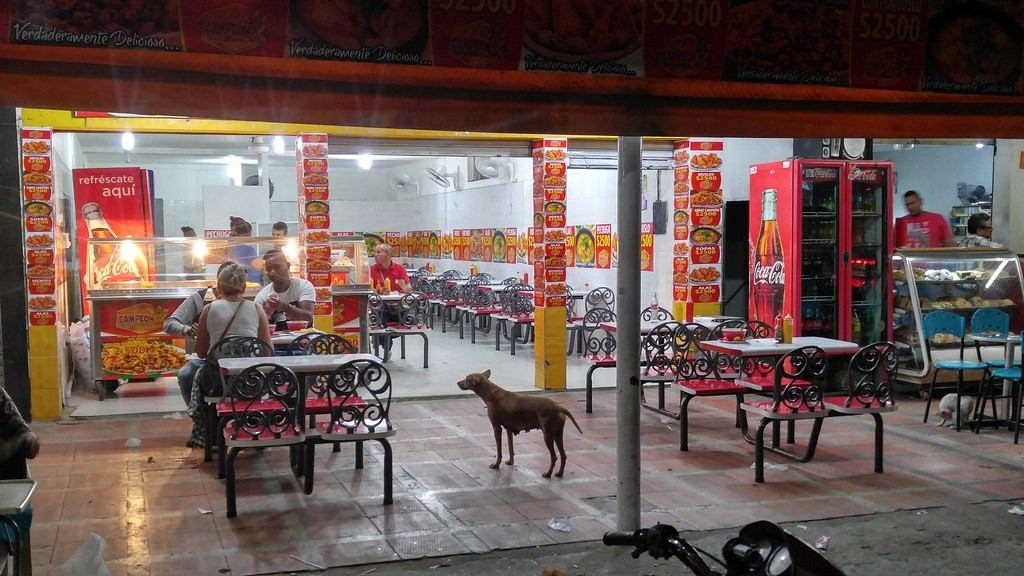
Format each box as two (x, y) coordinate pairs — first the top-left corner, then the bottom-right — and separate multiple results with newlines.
(100, 346), (185, 376)
(289, 331), (324, 336)
(718, 338), (747, 343)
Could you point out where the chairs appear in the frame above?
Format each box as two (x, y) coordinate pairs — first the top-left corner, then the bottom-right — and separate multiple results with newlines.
(197, 267), (899, 516)
(915, 306), (1023, 445)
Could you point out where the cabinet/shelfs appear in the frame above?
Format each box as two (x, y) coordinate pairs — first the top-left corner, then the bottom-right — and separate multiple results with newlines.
(892, 247), (1024, 387)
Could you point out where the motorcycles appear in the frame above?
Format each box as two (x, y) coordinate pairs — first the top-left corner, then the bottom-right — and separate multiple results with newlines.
(602, 520), (847, 576)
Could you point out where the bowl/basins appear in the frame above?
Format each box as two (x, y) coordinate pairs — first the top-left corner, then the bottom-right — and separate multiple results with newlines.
(426, 230), (528, 260)
(688, 158), (722, 283)
(543, 151), (566, 295)
(302, 148), (330, 246)
(721, 328), (747, 341)
(268, 324), (276, 336)
(912, 276), (1017, 302)
(286, 320), (309, 331)
(575, 227), (596, 264)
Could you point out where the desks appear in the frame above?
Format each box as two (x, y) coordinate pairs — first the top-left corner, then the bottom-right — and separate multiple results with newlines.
(964, 331), (1024, 433)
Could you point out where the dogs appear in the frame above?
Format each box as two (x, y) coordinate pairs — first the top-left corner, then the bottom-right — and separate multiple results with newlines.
(456, 368), (584, 479)
(932, 393), (976, 430)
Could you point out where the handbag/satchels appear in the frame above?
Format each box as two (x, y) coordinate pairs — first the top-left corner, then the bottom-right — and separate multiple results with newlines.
(198, 363), (223, 396)
(388, 300), (410, 315)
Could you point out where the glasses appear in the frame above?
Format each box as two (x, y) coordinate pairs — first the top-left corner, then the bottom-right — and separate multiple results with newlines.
(981, 225), (992, 231)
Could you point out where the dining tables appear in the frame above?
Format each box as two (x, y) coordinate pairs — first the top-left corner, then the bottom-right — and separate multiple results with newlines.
(699, 337), (858, 443)
(600, 315), (724, 410)
(218, 355), (385, 493)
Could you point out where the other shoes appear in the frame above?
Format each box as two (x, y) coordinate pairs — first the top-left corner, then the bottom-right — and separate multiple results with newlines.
(387, 350), (392, 361)
(372, 341), (379, 348)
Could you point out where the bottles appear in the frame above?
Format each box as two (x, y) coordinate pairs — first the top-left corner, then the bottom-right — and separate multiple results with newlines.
(802, 191), (874, 344)
(516, 272), (528, 285)
(675, 296), (694, 323)
(425, 261), (436, 274)
(470, 264), (477, 275)
(411, 259), (414, 269)
(370, 276), (390, 294)
(650, 293), (658, 323)
(82, 203), (144, 288)
(773, 312), (793, 344)
(752, 188), (785, 367)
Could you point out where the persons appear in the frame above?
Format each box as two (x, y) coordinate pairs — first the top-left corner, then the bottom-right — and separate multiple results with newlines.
(892, 191), (953, 330)
(953, 213), (1003, 298)
(253, 249), (315, 356)
(227, 216), (265, 282)
(195, 263), (274, 450)
(369, 243), (412, 359)
(272, 222), (300, 266)
(181, 227), (205, 280)
(163, 262), (234, 448)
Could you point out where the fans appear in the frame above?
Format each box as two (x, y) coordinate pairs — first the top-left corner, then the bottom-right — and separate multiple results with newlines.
(394, 175), (420, 194)
(245, 175), (274, 200)
(425, 166), (461, 190)
(475, 157), (516, 183)
